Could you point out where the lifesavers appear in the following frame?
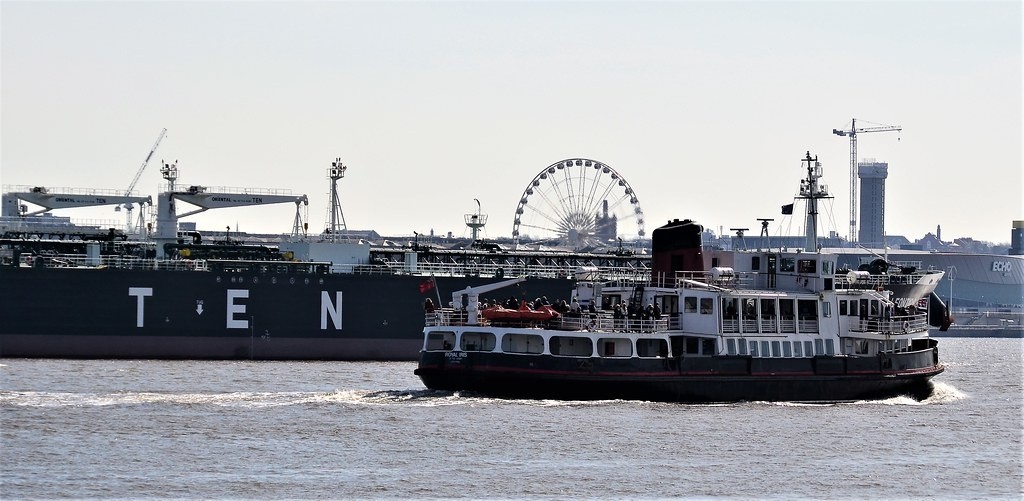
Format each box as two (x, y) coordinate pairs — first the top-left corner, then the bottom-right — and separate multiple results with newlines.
(903, 321), (910, 330)
(496, 268), (504, 279)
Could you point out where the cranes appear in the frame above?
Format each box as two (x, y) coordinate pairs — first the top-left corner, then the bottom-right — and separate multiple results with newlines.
(833, 118), (901, 248)
(115, 127), (169, 240)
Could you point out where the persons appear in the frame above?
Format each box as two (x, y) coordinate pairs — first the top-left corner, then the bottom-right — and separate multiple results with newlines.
(726, 300), (756, 319)
(894, 302), (916, 321)
(424, 296), (661, 333)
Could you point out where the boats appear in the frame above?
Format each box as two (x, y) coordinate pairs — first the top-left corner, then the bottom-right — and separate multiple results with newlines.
(413, 218), (955, 400)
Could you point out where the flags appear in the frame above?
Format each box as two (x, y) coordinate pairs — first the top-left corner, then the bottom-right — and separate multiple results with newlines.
(782, 203), (793, 215)
(420, 273), (437, 292)
